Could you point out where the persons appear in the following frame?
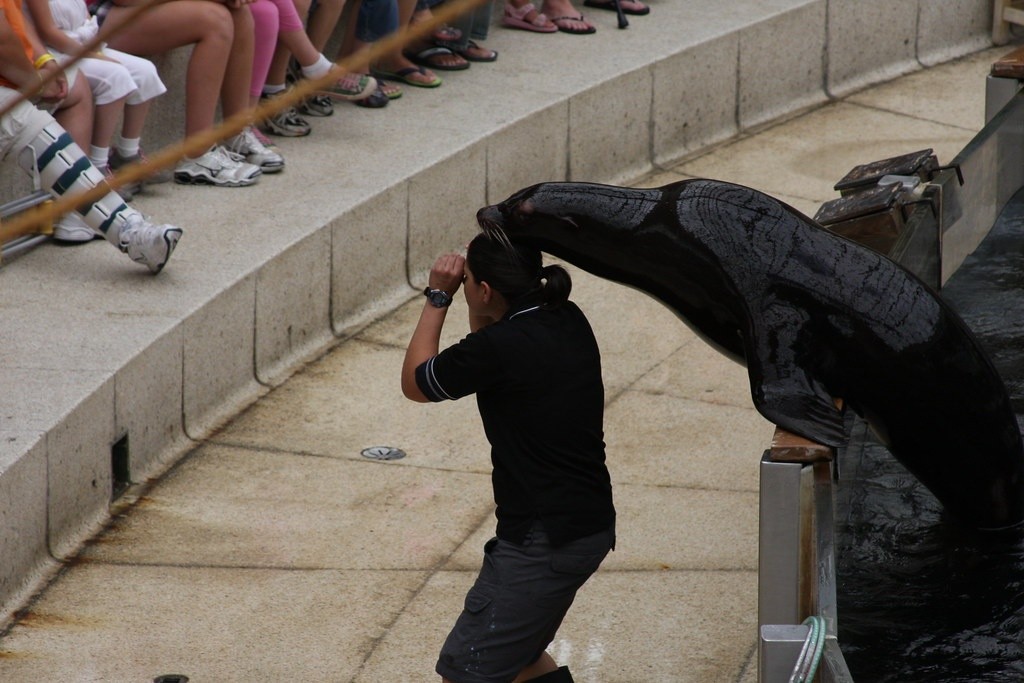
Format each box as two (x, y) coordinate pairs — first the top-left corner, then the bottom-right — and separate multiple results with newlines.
(401, 232), (618, 683)
(0, 0), (651, 275)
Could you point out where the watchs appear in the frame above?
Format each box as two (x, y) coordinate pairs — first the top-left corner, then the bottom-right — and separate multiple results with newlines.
(424, 287), (453, 308)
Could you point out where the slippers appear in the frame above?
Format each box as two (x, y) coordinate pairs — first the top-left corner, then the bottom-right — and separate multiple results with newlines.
(541, 5), (597, 34)
(373, 57), (442, 87)
(362, 73), (403, 98)
(433, 35), (498, 61)
(408, 18), (462, 38)
(402, 39), (470, 70)
(583, 0), (649, 15)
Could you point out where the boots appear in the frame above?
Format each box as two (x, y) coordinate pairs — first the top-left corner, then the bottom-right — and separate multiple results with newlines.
(12, 106), (183, 276)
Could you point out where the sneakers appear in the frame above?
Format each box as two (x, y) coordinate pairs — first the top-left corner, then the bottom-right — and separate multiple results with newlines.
(174, 141), (262, 186)
(50, 208), (104, 242)
(250, 122), (282, 156)
(256, 90), (312, 137)
(97, 166), (135, 201)
(107, 144), (172, 184)
(286, 64), (335, 116)
(303, 61), (376, 100)
(225, 123), (285, 173)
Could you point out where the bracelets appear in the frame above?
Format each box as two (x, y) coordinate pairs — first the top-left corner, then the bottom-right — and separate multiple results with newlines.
(37, 71), (45, 95)
(34, 53), (55, 70)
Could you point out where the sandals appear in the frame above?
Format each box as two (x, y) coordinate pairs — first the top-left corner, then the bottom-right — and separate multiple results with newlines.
(502, 1), (558, 32)
